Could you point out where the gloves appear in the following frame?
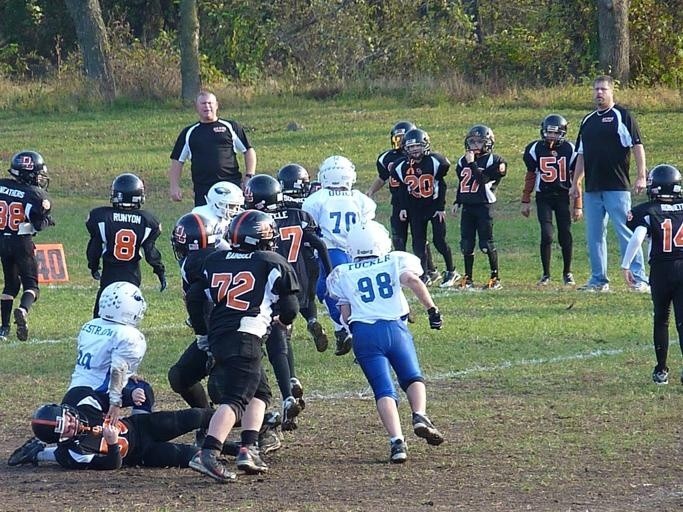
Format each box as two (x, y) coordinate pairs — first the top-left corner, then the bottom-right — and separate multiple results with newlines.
(427, 307), (442, 330)
(91, 267), (101, 280)
(158, 272), (167, 292)
(43, 214), (55, 227)
(195, 334), (215, 370)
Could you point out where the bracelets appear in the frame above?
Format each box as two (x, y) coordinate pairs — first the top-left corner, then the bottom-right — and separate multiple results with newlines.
(245, 174), (254, 179)
(573, 196), (583, 210)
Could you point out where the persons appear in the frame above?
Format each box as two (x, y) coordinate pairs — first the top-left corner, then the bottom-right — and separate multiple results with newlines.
(32, 402), (283, 471)
(169, 90), (257, 207)
(0, 150), (56, 342)
(364, 121), (443, 284)
(193, 180), (246, 243)
(324, 219), (446, 463)
(181, 209), (304, 484)
(243, 174), (333, 410)
(8, 279), (155, 466)
(568, 75), (651, 294)
(451, 124), (509, 291)
(167, 213), (284, 462)
(388, 129), (462, 288)
(520, 114), (583, 286)
(301, 155), (378, 355)
(619, 162), (682, 386)
(84, 173), (167, 319)
(277, 163), (319, 208)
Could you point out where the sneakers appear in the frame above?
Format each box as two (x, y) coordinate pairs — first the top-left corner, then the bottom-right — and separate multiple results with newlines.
(0, 326), (10, 341)
(8, 437), (46, 467)
(189, 449), (239, 483)
(390, 437), (409, 461)
(652, 365), (669, 385)
(291, 378), (305, 410)
(335, 328), (353, 356)
(576, 280), (609, 293)
(537, 276), (551, 286)
(412, 411), (443, 446)
(564, 273), (576, 287)
(439, 270), (461, 287)
(458, 279), (473, 290)
(627, 280), (651, 294)
(264, 412), (280, 429)
(429, 270), (443, 283)
(259, 431), (281, 454)
(237, 447), (268, 473)
(420, 274), (433, 287)
(483, 277), (503, 292)
(281, 397), (302, 430)
(14, 308), (29, 341)
(307, 321), (328, 352)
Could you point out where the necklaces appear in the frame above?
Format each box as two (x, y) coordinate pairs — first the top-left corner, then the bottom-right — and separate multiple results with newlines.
(597, 103), (615, 116)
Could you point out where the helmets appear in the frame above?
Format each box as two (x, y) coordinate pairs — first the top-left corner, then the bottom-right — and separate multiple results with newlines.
(401, 128), (431, 159)
(204, 181), (244, 220)
(230, 209), (280, 252)
(390, 121), (416, 151)
(646, 164), (682, 199)
(171, 212), (214, 260)
(110, 174), (146, 210)
(97, 281), (147, 325)
(33, 403), (89, 444)
(465, 125), (495, 154)
(318, 156), (356, 191)
(8, 151), (50, 192)
(541, 114), (567, 149)
(245, 174), (284, 213)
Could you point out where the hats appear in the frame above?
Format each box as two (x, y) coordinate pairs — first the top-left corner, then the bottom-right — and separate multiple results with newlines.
(346, 220), (392, 262)
(278, 164), (311, 198)
